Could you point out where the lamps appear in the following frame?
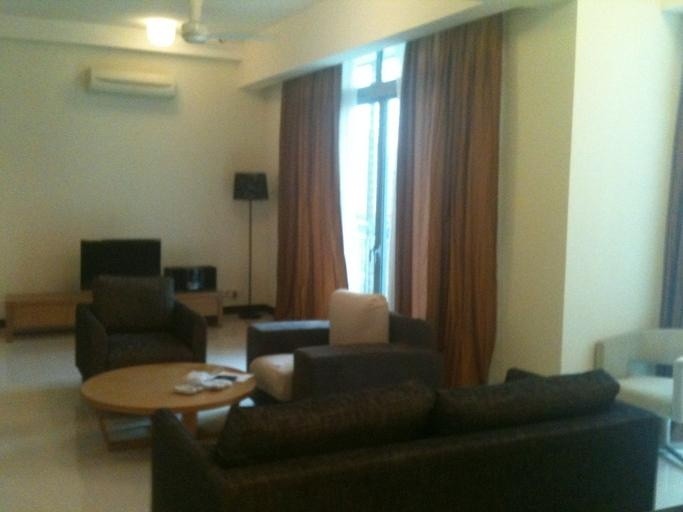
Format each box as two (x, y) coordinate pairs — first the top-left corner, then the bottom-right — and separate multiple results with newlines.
(231, 171), (270, 320)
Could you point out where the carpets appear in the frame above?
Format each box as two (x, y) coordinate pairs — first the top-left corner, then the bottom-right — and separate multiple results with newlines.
(0, 383), (242, 512)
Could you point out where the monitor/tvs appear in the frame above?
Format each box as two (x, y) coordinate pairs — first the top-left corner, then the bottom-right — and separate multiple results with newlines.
(80, 239), (162, 290)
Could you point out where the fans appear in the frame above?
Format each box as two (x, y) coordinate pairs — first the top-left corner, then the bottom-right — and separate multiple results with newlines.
(171, 1), (270, 48)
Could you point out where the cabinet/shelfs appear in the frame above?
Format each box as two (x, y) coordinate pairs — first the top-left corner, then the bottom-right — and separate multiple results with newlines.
(2, 292), (224, 342)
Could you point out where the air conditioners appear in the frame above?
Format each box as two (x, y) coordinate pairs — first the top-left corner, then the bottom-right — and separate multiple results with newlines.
(84, 66), (176, 100)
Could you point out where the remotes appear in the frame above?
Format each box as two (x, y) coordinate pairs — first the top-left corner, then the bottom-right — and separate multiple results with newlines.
(216, 374), (237, 380)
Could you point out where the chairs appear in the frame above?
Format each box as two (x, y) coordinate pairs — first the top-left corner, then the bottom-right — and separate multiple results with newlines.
(73, 274), (209, 381)
(246, 287), (441, 405)
(593, 325), (682, 464)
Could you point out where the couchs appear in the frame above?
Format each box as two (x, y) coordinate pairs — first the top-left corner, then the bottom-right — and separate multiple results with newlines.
(151, 367), (662, 511)
